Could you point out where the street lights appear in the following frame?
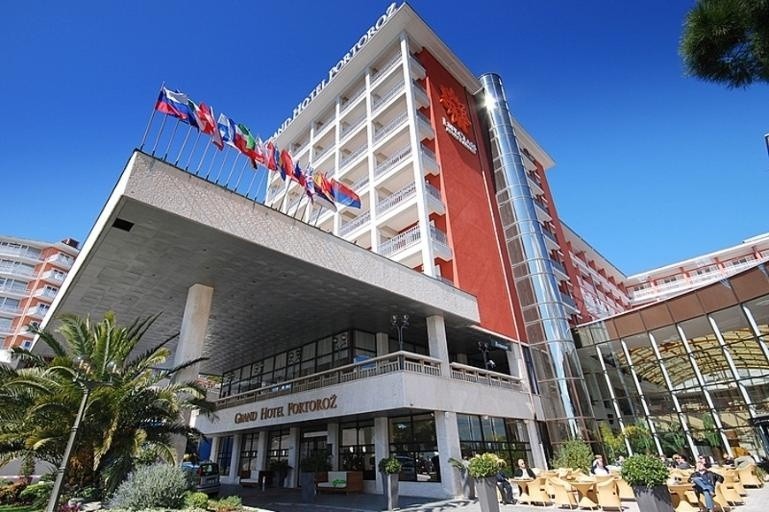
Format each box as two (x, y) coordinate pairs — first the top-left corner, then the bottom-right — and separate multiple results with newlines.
(43, 350), (124, 511)
(390, 314), (411, 370)
(476, 341), (491, 371)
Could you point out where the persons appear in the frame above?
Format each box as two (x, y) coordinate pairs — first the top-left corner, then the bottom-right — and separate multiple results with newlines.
(514, 457), (536, 480)
(496, 470), (519, 504)
(591, 453), (608, 474)
(617, 444), (769, 486)
(688, 459), (725, 512)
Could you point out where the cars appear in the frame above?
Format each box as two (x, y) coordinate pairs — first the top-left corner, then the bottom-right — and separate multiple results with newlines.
(393, 454), (433, 477)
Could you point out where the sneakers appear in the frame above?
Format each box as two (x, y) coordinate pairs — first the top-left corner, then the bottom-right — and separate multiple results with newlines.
(502, 501), (514, 505)
(709, 509), (714, 512)
(710, 492), (716, 497)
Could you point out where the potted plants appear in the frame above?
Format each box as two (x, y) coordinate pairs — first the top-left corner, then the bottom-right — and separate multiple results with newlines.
(377, 456), (402, 511)
(467, 452), (507, 512)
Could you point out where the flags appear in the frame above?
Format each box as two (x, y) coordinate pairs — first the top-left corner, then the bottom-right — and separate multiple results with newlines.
(155, 86), (360, 214)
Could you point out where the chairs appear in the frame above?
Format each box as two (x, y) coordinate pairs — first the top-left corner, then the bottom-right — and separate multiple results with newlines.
(509, 458), (763, 512)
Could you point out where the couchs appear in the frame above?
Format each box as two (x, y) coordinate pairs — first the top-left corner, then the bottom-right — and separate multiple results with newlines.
(315, 471), (365, 497)
(239, 471), (262, 490)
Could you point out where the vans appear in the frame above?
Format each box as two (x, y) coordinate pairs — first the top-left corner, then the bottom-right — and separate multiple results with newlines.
(181, 461), (222, 499)
